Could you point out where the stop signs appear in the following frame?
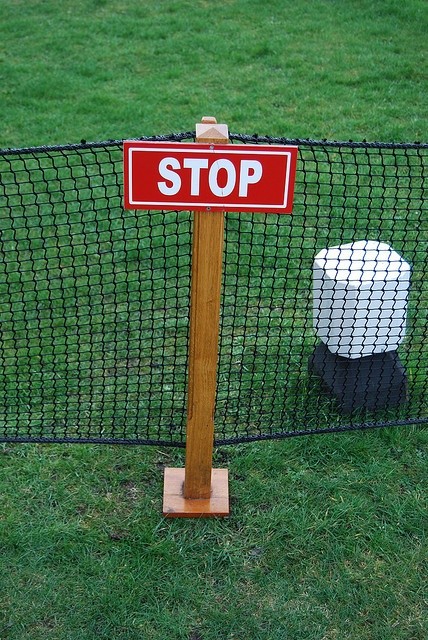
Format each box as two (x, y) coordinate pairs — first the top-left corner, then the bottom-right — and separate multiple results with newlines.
(122, 140), (298, 216)
